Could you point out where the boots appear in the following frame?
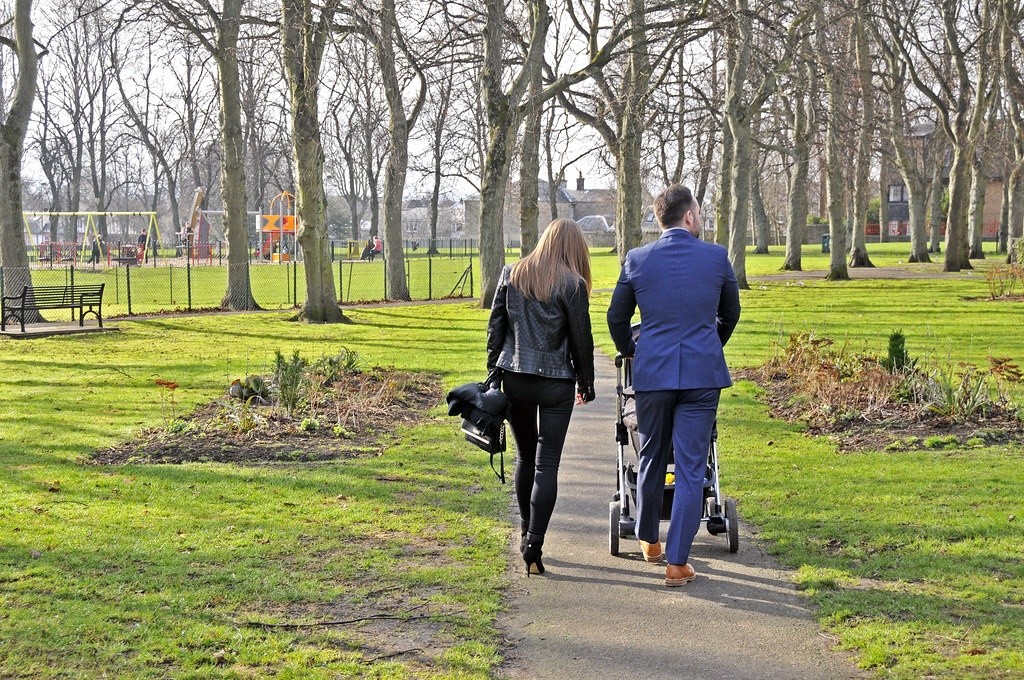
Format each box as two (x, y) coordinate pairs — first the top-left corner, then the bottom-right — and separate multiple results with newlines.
(522, 532), (545, 578)
(519, 518), (529, 553)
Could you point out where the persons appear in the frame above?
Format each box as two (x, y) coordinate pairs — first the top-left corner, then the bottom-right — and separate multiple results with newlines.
(87, 234), (105, 264)
(257, 241), (299, 260)
(607, 185), (741, 587)
(360, 236), (382, 260)
(507, 240), (512, 253)
(138, 230), (150, 264)
(486, 218), (596, 576)
(181, 222), (193, 242)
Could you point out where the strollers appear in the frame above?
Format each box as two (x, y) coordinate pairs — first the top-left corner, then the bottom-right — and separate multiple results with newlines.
(607, 322), (740, 554)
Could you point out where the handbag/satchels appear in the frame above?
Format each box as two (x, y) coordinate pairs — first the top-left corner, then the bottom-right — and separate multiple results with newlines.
(461, 372), (507, 484)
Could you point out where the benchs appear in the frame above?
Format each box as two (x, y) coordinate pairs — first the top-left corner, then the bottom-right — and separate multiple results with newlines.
(2, 283), (106, 332)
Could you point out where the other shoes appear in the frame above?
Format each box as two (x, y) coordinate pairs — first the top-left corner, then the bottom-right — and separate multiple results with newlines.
(638, 539), (663, 562)
(665, 564), (697, 587)
